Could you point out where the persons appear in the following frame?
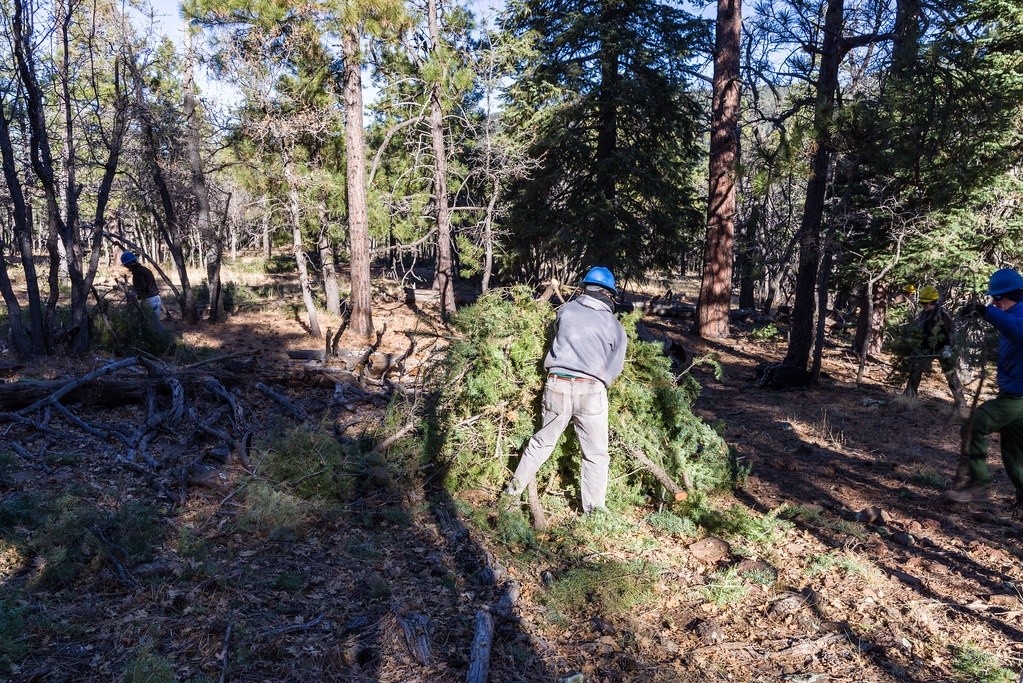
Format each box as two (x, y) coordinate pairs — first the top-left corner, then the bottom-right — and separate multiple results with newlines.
(498, 264), (628, 522)
(944, 268), (1023, 520)
(900, 284), (967, 411)
(113, 251), (162, 325)
(879, 284), (917, 354)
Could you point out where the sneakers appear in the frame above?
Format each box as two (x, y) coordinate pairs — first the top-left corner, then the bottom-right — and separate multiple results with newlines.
(947, 483), (994, 504)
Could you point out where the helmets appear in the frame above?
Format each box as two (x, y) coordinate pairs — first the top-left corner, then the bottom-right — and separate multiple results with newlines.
(989, 270), (1023, 295)
(919, 286), (939, 302)
(901, 284), (916, 293)
(121, 252), (137, 265)
(577, 267), (619, 296)
